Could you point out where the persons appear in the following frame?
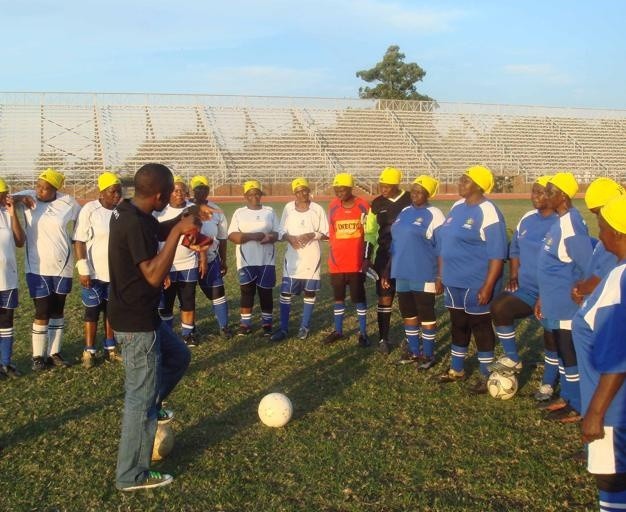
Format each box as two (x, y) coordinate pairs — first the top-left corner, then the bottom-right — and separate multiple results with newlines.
(151, 175), (207, 348)
(323, 172), (370, 348)
(108, 163), (224, 492)
(572, 176), (618, 305)
(382, 175), (448, 371)
(190, 175), (232, 342)
(536, 172), (593, 424)
(270, 177), (330, 341)
(570, 193), (625, 508)
(0, 180), (27, 383)
(439, 164), (509, 394)
(486, 175), (561, 403)
(74, 171), (131, 370)
(227, 180), (280, 338)
(363, 166), (413, 345)
(8, 168), (83, 372)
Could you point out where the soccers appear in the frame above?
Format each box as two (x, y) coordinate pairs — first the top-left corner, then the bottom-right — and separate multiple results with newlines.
(258, 393), (292, 428)
(487, 372), (518, 401)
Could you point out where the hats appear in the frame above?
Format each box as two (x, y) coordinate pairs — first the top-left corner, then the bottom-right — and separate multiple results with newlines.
(463, 166), (494, 195)
(0, 178), (9, 192)
(534, 176), (554, 187)
(39, 168), (64, 190)
(191, 176), (209, 190)
(292, 178), (308, 191)
(174, 175), (185, 184)
(413, 175), (438, 198)
(333, 173), (353, 187)
(97, 172), (122, 192)
(547, 172), (578, 199)
(585, 178), (626, 209)
(378, 167), (403, 185)
(600, 193), (626, 235)
(244, 181), (261, 193)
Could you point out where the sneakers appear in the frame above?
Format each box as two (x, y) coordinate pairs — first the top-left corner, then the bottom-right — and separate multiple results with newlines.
(270, 330), (287, 341)
(158, 410), (173, 424)
(359, 335), (371, 345)
(417, 355), (435, 371)
(3, 365), (22, 378)
(219, 328), (231, 339)
(30, 357), (44, 372)
(431, 369), (466, 382)
(471, 381), (487, 394)
(488, 355), (523, 372)
(297, 325), (310, 340)
(380, 340), (390, 356)
(47, 352), (71, 370)
(81, 351), (95, 369)
(120, 471), (173, 489)
(0, 367), (9, 381)
(323, 331), (336, 345)
(183, 335), (197, 347)
(534, 383), (553, 400)
(238, 328), (253, 337)
(104, 350), (123, 363)
(263, 329), (271, 337)
(398, 352), (420, 365)
